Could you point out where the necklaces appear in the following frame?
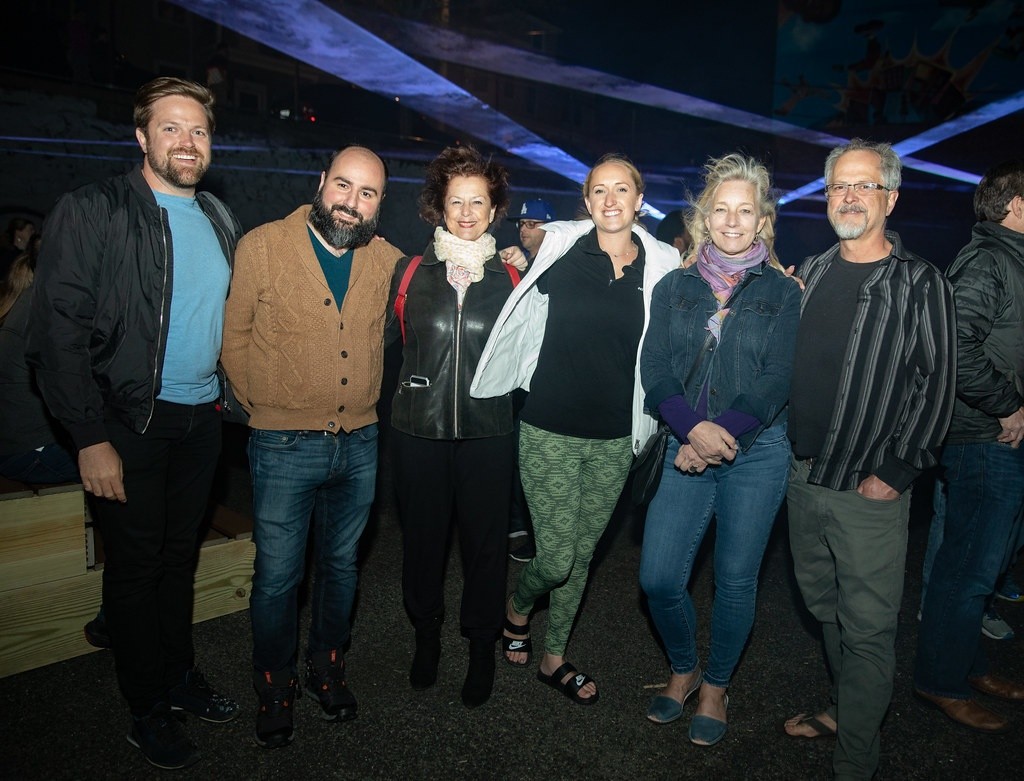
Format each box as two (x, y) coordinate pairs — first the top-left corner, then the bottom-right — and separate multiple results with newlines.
(599, 241), (636, 257)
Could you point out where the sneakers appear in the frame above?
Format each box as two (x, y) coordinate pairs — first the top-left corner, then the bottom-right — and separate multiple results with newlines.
(170, 665), (238, 725)
(126, 712), (198, 769)
(982, 605), (1015, 640)
(307, 651), (360, 720)
(995, 582), (1024, 601)
(253, 672), (298, 748)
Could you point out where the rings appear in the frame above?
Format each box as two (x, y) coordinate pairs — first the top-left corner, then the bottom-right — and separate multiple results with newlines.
(690, 466), (697, 471)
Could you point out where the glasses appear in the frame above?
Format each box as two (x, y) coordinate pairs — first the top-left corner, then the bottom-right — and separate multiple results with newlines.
(825, 182), (890, 197)
(515, 220), (545, 229)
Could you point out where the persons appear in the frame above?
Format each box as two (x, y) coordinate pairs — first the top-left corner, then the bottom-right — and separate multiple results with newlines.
(784, 138), (957, 781)
(384, 144), (524, 707)
(27, 77), (242, 770)
(657, 210), (695, 255)
(219, 142), (529, 747)
(0, 218), (41, 324)
(912, 172), (1024, 733)
(470, 147), (806, 706)
(640, 156), (802, 746)
(506, 196), (554, 266)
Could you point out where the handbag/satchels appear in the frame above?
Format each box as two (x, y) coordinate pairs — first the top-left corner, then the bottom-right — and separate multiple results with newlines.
(620, 432), (669, 520)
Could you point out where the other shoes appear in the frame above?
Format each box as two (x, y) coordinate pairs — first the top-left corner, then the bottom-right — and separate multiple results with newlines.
(409, 627), (441, 691)
(84, 604), (113, 649)
(509, 540), (535, 562)
(463, 643), (497, 708)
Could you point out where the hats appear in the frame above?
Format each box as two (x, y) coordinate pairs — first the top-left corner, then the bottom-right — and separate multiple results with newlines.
(506, 197), (557, 223)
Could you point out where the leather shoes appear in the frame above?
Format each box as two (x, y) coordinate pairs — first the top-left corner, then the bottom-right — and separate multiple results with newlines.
(912, 687), (1010, 734)
(973, 673), (1024, 702)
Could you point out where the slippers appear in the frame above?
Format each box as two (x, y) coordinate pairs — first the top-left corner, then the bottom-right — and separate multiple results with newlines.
(537, 662), (599, 704)
(775, 710), (837, 739)
(688, 692), (730, 746)
(503, 593), (533, 668)
(648, 668), (703, 723)
